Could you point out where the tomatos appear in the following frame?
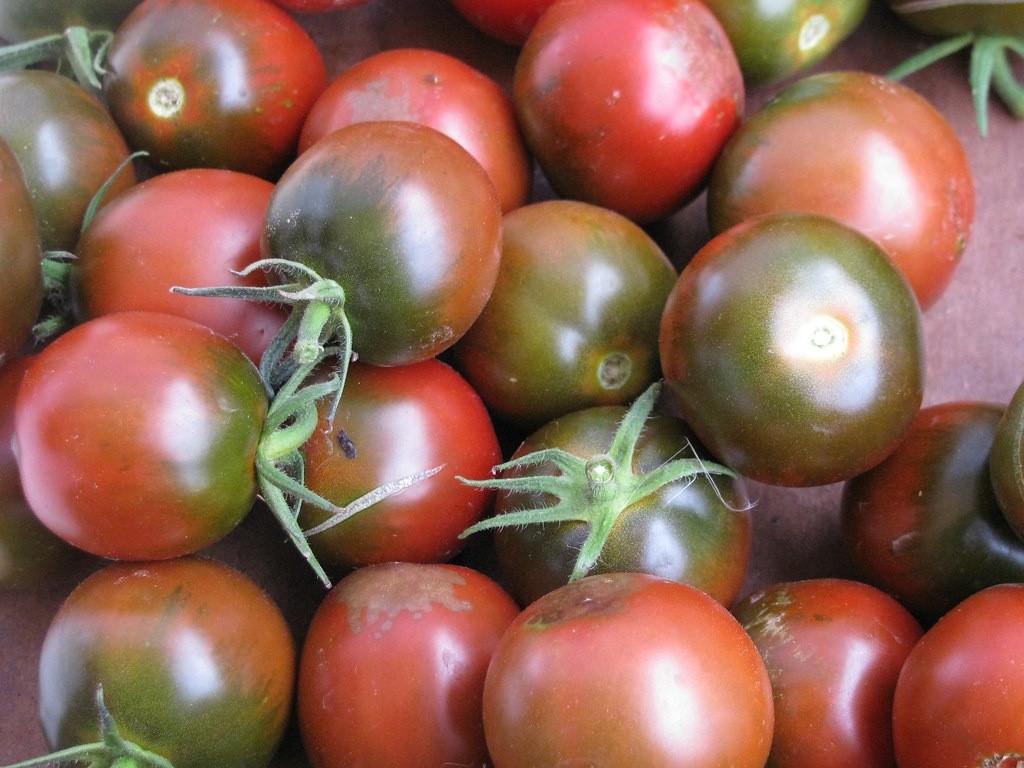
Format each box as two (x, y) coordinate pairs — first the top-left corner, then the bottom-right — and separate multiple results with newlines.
(0, 0), (1024, 767)
